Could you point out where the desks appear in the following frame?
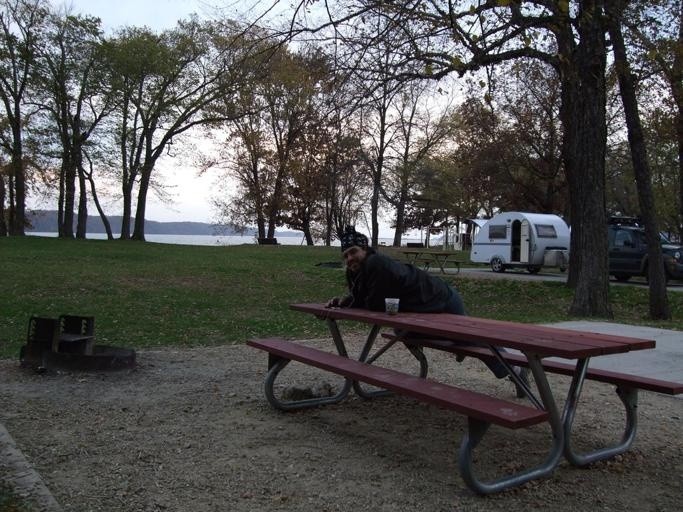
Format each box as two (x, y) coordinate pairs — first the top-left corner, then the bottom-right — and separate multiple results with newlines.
(289, 301), (657, 462)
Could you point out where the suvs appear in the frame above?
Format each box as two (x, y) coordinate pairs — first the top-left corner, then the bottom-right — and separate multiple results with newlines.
(607, 216), (682, 285)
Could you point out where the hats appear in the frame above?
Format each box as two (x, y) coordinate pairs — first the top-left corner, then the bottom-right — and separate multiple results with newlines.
(341, 225), (368, 251)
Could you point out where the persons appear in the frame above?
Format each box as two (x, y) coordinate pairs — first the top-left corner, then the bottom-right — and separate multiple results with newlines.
(324, 231), (532, 397)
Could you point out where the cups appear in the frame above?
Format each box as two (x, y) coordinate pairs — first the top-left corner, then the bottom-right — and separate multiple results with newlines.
(384, 298), (400, 315)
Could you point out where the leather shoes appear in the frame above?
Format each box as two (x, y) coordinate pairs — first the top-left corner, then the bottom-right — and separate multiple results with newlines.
(508, 366), (531, 398)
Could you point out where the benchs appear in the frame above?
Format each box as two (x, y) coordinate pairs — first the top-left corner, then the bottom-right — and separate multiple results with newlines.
(400, 251), (465, 277)
(247, 337), (548, 497)
(376, 331), (683, 467)
